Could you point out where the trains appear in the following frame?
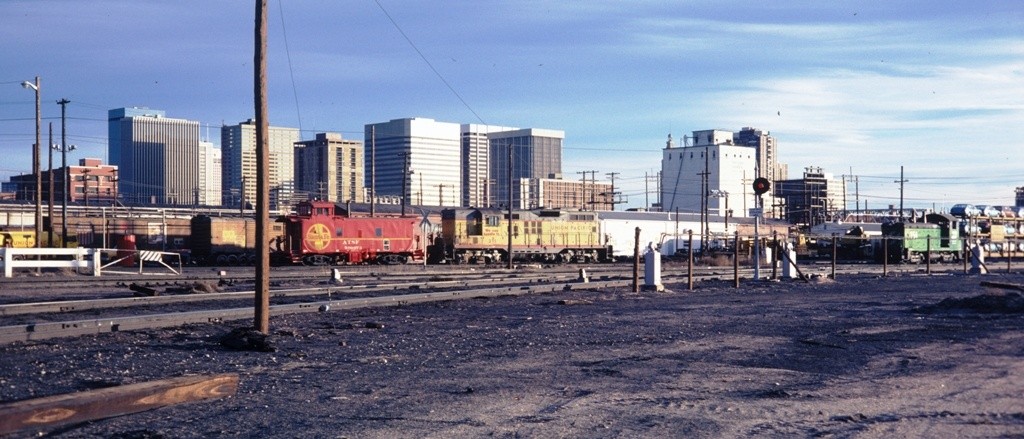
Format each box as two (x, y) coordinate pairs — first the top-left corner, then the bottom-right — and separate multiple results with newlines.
(284, 197), (434, 266)
(889, 204), (1024, 264)
(439, 206), (619, 264)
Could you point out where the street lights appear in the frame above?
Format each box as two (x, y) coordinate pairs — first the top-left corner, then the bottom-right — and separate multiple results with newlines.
(21, 75), (43, 276)
(52, 143), (78, 248)
